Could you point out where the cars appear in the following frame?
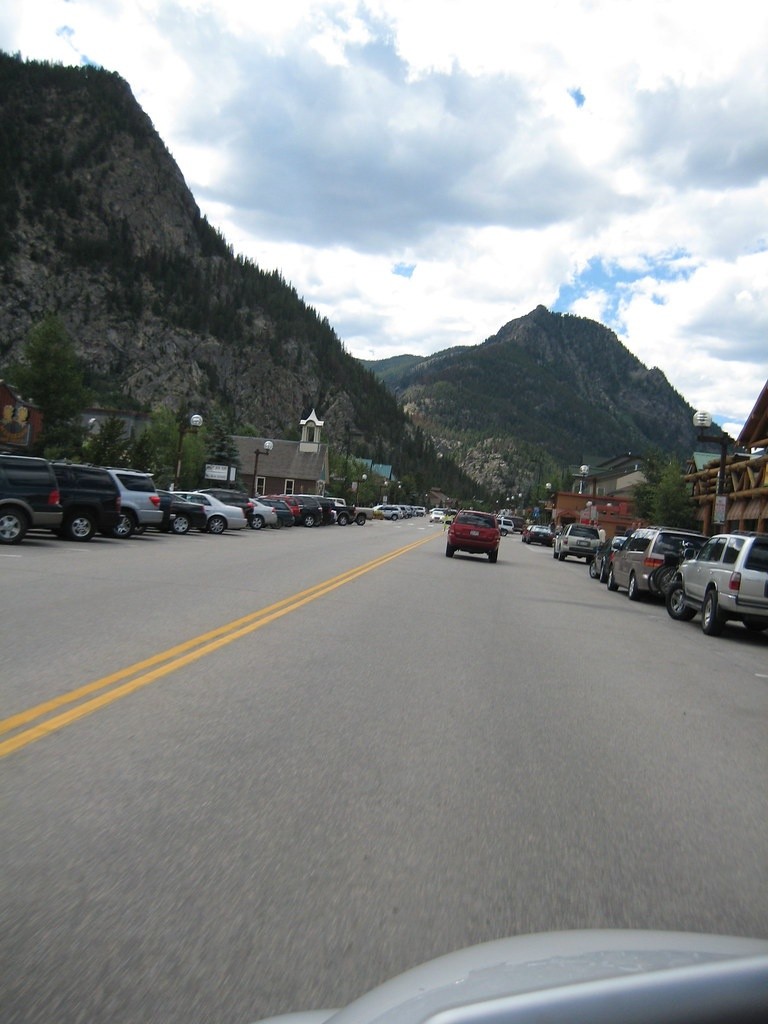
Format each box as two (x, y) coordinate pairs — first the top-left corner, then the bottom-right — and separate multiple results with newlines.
(157, 487), (209, 536)
(247, 497), (278, 530)
(521, 524), (553, 548)
(168, 490), (249, 535)
(429, 510), (445, 523)
(589, 536), (628, 582)
(443, 509), (458, 524)
(495, 516), (515, 537)
(445, 509), (500, 564)
(255, 497), (294, 530)
(262, 493), (442, 532)
(204, 488), (254, 526)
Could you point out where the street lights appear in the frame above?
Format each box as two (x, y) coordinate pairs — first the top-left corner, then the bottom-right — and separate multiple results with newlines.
(377, 481), (390, 505)
(173, 414), (205, 490)
(579, 465), (595, 529)
(529, 457), (543, 506)
(250, 440), (274, 498)
(693, 409), (730, 535)
(353, 473), (368, 508)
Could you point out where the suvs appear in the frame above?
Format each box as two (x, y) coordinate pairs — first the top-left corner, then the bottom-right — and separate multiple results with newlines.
(0, 453), (66, 545)
(103, 465), (165, 538)
(554, 523), (602, 561)
(46, 459), (126, 542)
(665, 530), (768, 638)
(606, 526), (711, 601)
